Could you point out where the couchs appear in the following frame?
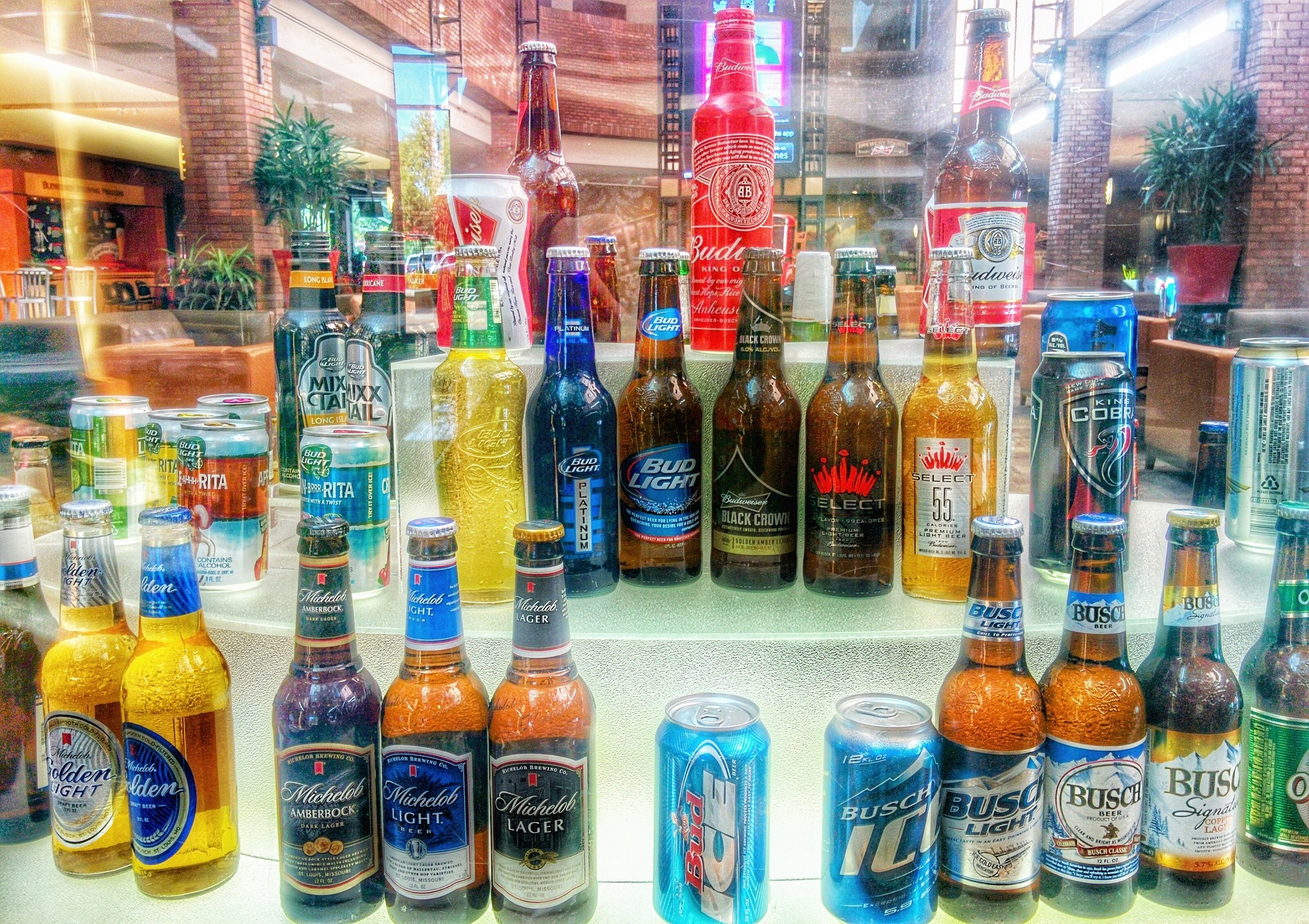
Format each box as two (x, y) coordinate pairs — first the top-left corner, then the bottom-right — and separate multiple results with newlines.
(0, 310), (276, 426)
(1144, 309), (1308, 474)
(1021, 289), (1167, 406)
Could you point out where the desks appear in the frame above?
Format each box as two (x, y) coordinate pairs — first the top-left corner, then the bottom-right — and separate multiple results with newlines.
(1, 270), (53, 318)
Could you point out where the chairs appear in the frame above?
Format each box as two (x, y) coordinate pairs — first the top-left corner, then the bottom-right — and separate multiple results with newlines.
(112, 281), (142, 311)
(99, 282), (117, 312)
(135, 280), (154, 310)
(6, 267), (50, 318)
(138, 284), (157, 309)
(51, 267), (97, 316)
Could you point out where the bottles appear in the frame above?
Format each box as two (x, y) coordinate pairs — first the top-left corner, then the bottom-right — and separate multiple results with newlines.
(0, 0), (1306, 924)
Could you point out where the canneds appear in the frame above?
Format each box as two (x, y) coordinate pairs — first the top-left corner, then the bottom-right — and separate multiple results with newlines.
(1036, 284), (1140, 380)
(297, 423), (391, 601)
(65, 389), (150, 539)
(193, 389), (270, 432)
(1220, 334), (1308, 551)
(425, 171), (532, 357)
(1028, 350), (1140, 591)
(141, 409), (220, 509)
(820, 686), (949, 922)
(653, 691), (766, 924)
(167, 419), (271, 594)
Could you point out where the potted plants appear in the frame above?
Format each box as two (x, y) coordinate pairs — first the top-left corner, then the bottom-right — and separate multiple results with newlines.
(1131, 80), (1296, 305)
(241, 95), (377, 258)
